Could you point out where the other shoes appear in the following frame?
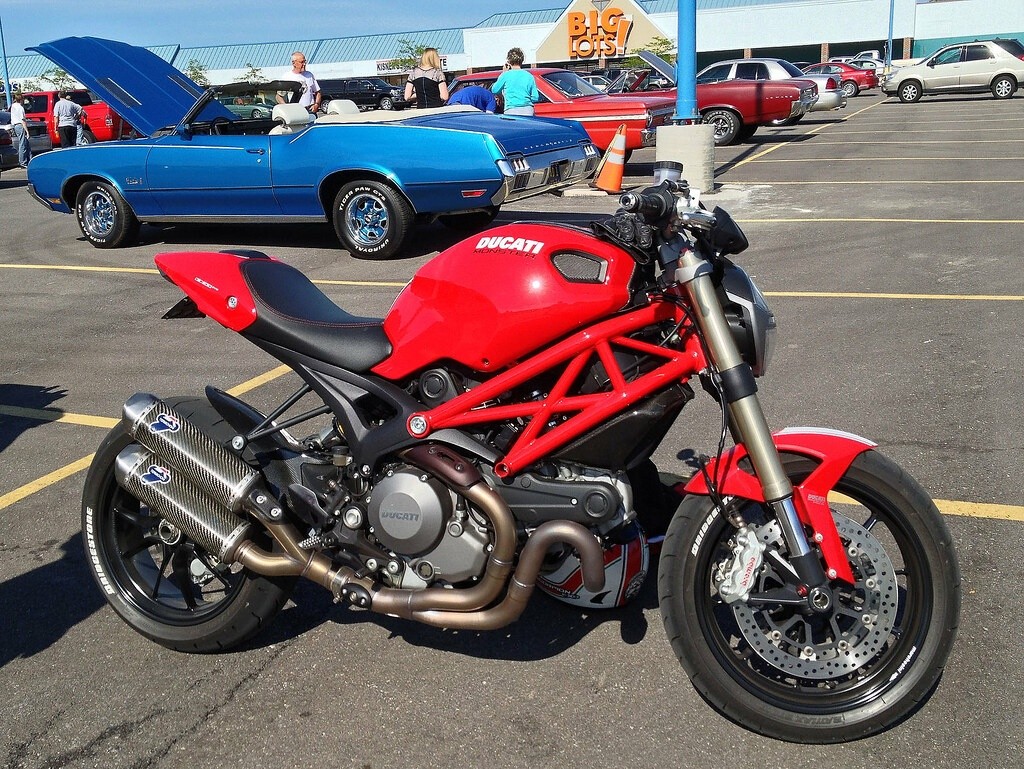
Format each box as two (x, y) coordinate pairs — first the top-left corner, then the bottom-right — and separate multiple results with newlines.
(21, 166), (27, 169)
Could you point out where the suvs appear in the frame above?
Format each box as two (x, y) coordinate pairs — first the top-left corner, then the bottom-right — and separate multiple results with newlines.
(881, 37), (1024, 104)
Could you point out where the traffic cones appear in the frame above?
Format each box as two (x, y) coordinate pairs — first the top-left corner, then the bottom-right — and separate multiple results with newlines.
(592, 124), (629, 195)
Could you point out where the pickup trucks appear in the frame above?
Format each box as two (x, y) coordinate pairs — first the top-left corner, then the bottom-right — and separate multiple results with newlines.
(313, 77), (416, 114)
(5, 90), (142, 147)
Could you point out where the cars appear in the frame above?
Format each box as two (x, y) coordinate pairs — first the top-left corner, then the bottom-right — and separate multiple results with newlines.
(601, 50), (819, 147)
(576, 76), (618, 94)
(25, 36), (599, 261)
(0, 110), (53, 156)
(801, 62), (879, 97)
(220, 98), (274, 120)
(829, 50), (904, 87)
(696, 58), (848, 127)
(443, 68), (701, 163)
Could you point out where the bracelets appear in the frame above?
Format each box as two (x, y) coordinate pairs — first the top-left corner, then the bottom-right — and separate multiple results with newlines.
(317, 103), (319, 107)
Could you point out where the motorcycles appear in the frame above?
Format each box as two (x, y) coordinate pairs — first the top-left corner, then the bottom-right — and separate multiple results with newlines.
(82, 160), (960, 746)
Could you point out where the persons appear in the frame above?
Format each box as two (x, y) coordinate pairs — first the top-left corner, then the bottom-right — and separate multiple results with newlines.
(492, 48), (539, 116)
(11, 92), (33, 169)
(53, 92), (81, 149)
(447, 86), (503, 115)
(66, 95), (88, 146)
(404, 48), (449, 109)
(275, 52), (321, 119)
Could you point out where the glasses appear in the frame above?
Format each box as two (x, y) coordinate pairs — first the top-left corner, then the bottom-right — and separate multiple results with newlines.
(240, 103), (244, 105)
(296, 59), (307, 64)
